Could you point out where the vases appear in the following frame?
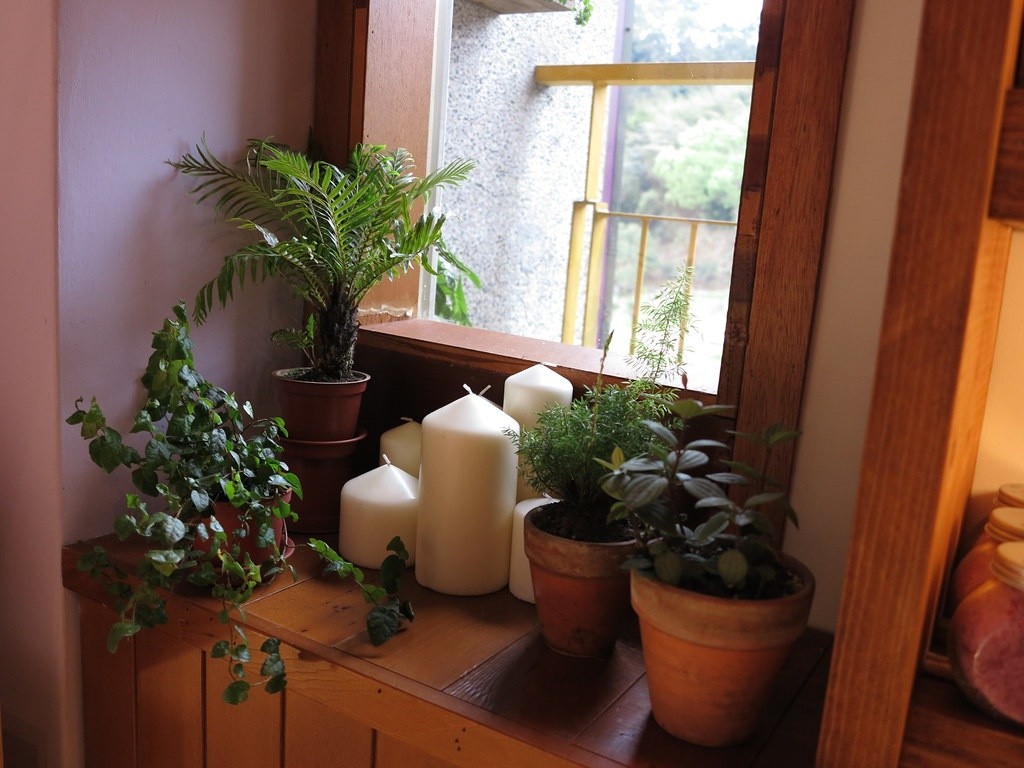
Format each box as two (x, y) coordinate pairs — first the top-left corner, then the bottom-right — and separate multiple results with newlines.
(266, 432), (371, 546)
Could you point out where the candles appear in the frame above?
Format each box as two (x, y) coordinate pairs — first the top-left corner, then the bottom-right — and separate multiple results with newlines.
(377, 413), (423, 481)
(508, 489), (565, 605)
(415, 382), (525, 599)
(501, 361), (575, 501)
(338, 451), (420, 571)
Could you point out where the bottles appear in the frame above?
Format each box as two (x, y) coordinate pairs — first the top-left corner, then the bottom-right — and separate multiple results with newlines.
(967, 483), (1024, 552)
(947, 542), (1024, 732)
(951, 507), (1024, 613)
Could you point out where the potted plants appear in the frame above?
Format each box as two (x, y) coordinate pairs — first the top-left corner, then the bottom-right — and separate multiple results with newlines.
(63, 296), (418, 711)
(503, 380), (685, 662)
(598, 398), (820, 749)
(163, 129), (482, 441)
